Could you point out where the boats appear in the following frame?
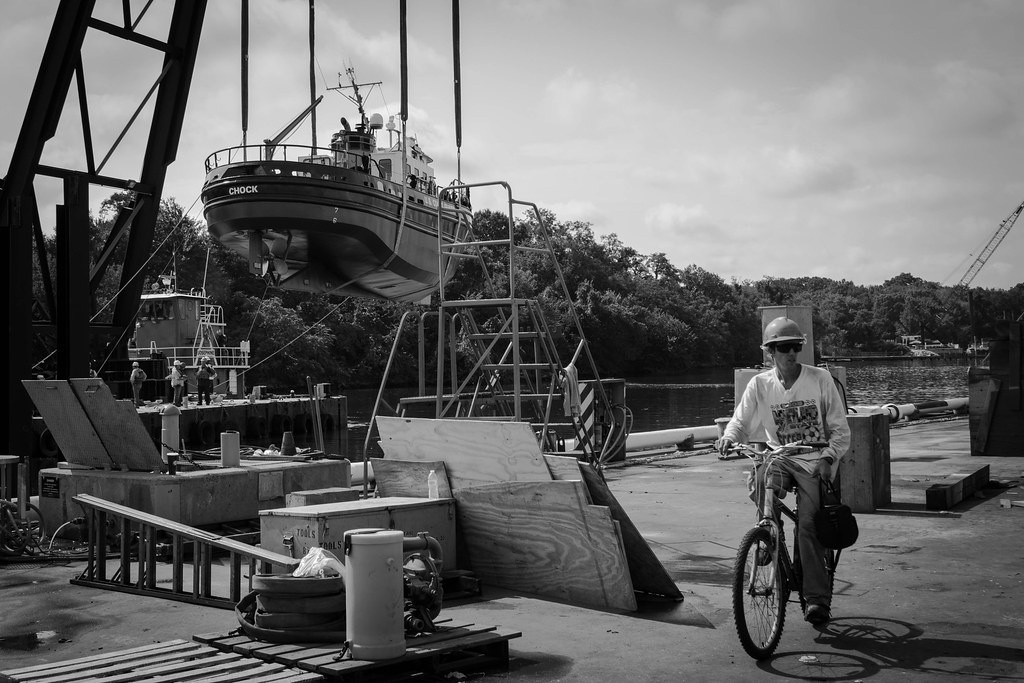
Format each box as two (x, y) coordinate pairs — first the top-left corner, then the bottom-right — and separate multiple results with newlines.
(199, 68), (473, 306)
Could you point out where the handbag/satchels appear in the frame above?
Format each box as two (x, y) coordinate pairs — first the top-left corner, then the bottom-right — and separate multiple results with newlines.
(819, 474), (859, 549)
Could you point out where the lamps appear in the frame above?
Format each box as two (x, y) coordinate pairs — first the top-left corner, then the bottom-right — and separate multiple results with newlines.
(125, 180), (137, 189)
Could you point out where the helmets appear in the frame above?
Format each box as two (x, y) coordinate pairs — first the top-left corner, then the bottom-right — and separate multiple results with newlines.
(131, 361), (139, 367)
(205, 356), (210, 360)
(173, 359), (181, 365)
(180, 362), (186, 368)
(762, 317), (806, 344)
(200, 357), (207, 362)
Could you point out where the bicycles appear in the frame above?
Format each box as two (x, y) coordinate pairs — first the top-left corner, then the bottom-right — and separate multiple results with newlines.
(712, 439), (840, 659)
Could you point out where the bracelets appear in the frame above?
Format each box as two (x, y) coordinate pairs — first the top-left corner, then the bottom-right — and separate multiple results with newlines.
(823, 457), (833, 465)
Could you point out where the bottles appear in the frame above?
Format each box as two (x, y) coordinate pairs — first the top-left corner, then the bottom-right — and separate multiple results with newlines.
(428, 470), (440, 500)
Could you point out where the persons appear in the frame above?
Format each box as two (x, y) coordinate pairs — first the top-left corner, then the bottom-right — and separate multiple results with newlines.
(130, 362), (147, 403)
(171, 360), (186, 405)
(194, 357), (218, 405)
(714, 316), (851, 628)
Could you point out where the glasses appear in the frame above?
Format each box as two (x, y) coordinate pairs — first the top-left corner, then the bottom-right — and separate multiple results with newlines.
(769, 343), (804, 353)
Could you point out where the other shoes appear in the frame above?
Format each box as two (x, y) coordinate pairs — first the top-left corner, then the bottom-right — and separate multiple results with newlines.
(206, 402), (209, 405)
(803, 600), (830, 629)
(139, 404), (145, 406)
(134, 406), (139, 408)
(754, 532), (786, 566)
(177, 404), (183, 407)
(197, 402), (202, 405)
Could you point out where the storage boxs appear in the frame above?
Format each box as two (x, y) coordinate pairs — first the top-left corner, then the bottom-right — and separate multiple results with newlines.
(258, 496), (457, 599)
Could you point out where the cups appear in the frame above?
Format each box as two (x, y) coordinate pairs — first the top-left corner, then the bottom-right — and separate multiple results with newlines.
(167, 452), (179, 474)
